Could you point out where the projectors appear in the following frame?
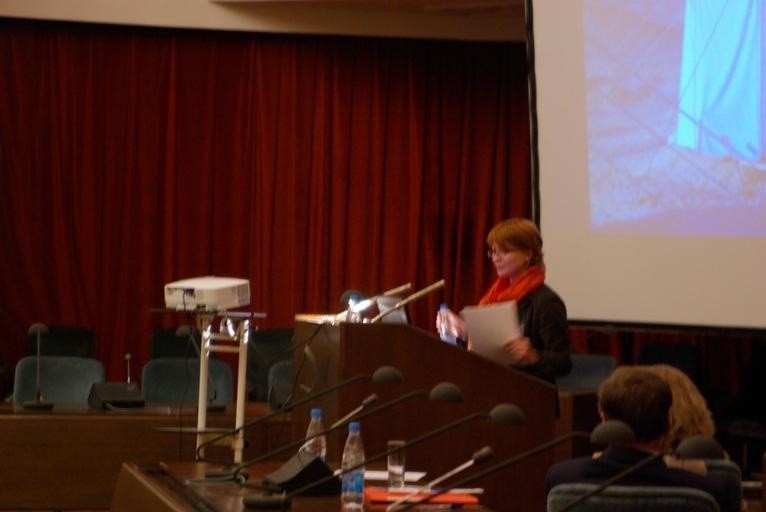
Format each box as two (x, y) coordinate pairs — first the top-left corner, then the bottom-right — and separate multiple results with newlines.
(163, 276), (251, 312)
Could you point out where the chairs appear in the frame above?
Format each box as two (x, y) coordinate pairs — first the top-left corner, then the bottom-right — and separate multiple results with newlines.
(547, 353), (746, 511)
(11, 324), (294, 411)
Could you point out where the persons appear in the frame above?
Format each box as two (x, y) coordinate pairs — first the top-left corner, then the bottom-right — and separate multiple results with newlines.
(540, 367), (739, 512)
(592, 361), (749, 512)
(435, 216), (574, 421)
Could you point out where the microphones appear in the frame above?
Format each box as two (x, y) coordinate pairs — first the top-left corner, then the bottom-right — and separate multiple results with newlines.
(231, 381), (463, 489)
(333, 394), (379, 426)
(279, 404), (524, 512)
(561, 435), (725, 512)
(336, 282), (412, 318)
(385, 445), (492, 512)
(194, 364), (402, 460)
(124, 354), (133, 385)
(370, 280), (445, 323)
(392, 420), (639, 511)
(23, 322), (54, 411)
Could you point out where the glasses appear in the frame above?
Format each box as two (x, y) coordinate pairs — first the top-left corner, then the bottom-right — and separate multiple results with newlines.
(487, 248), (522, 258)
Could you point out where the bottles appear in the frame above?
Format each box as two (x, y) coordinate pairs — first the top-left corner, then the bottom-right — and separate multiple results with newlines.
(341, 423), (365, 508)
(440, 303), (456, 345)
(345, 293), (360, 324)
(305, 407), (325, 458)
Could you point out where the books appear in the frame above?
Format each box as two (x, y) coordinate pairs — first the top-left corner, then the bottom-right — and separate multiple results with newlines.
(333, 466), (429, 482)
(363, 484), (480, 504)
(460, 299), (523, 367)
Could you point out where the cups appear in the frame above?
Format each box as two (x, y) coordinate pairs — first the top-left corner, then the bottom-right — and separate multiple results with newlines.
(387, 439), (406, 495)
